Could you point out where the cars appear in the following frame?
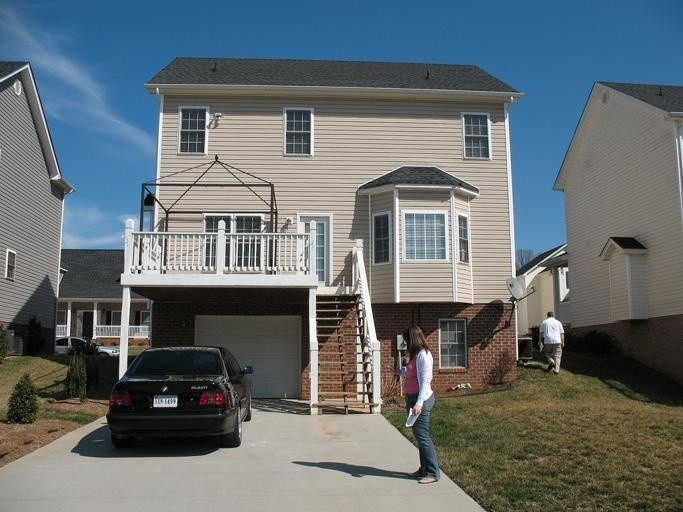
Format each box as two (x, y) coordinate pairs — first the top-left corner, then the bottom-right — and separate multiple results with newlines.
(54, 335), (120, 359)
(102, 343), (256, 451)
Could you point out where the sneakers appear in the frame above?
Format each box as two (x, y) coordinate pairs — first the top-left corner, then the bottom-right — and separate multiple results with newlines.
(548, 362), (559, 375)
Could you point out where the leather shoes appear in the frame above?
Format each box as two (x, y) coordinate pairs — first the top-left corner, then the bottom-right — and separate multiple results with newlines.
(408, 470), (425, 477)
(419, 476), (438, 483)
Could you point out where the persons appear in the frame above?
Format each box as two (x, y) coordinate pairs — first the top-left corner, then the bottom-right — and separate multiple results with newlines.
(536, 310), (565, 377)
(399, 323), (439, 484)
